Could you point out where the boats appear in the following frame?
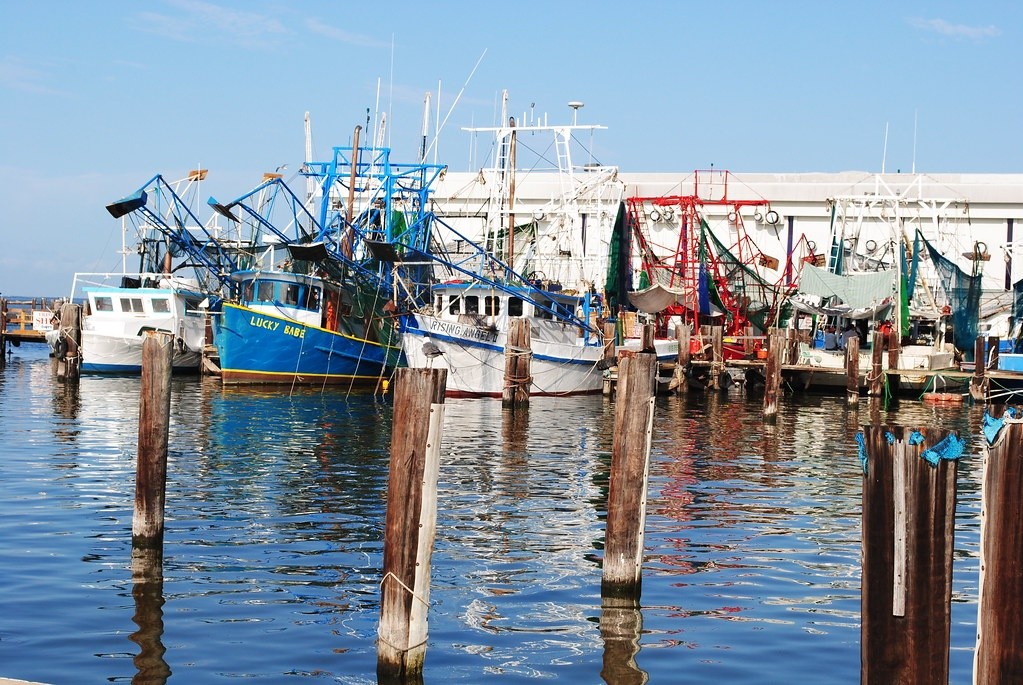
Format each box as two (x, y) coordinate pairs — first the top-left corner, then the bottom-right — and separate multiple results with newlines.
(44, 33), (954, 397)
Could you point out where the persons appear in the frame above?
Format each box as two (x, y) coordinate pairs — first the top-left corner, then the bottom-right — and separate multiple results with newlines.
(876, 319), (892, 348)
(825, 323), (861, 351)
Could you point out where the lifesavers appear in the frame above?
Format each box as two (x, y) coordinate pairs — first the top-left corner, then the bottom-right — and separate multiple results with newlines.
(923, 393), (963, 401)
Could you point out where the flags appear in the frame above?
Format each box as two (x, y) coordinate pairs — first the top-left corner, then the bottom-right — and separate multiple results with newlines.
(262, 173), (283, 185)
(188, 170), (208, 181)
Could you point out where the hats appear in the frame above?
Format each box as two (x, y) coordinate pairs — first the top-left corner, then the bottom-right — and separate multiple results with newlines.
(828, 328), (836, 332)
